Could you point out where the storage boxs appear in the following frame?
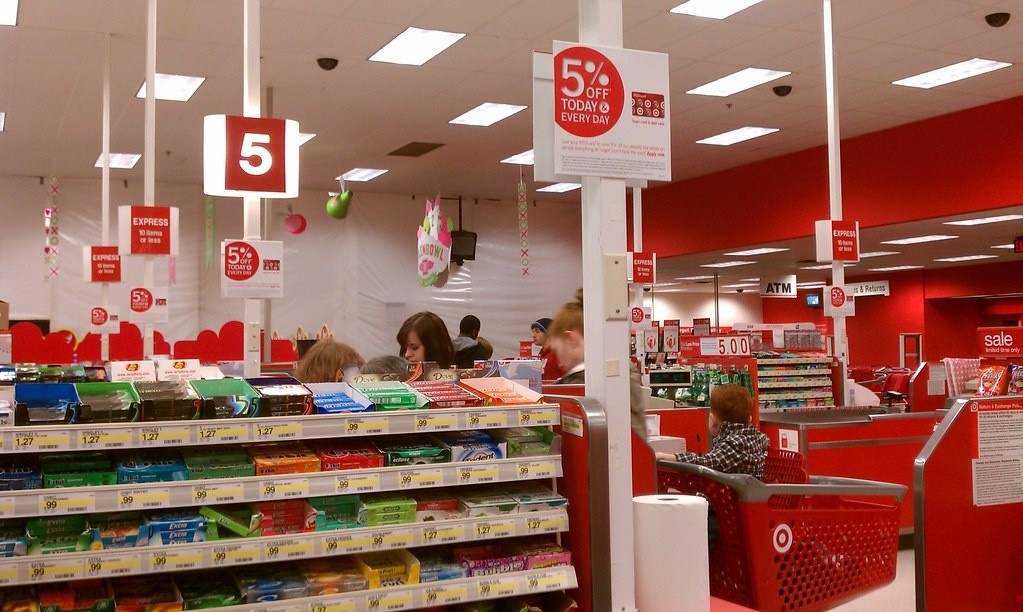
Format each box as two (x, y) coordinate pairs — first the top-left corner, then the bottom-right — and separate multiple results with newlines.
(1, 539), (571, 612)
(1, 402), (580, 612)
(0, 482), (567, 555)
(0, 377), (545, 427)
(104, 359), (202, 383)
(0, 429), (560, 492)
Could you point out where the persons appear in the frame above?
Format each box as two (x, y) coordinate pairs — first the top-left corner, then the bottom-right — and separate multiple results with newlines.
(294, 338), (365, 383)
(531, 318), (568, 380)
(452, 314), (489, 369)
(360, 354), (411, 375)
(655, 384), (768, 497)
(395, 311), (456, 370)
(548, 287), (648, 443)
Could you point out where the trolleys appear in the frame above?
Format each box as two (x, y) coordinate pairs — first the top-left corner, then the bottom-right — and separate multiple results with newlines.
(656, 458), (907, 612)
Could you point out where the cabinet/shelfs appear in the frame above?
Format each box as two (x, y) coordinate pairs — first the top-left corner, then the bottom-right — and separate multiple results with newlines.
(755, 356), (834, 400)
(0, 380), (598, 612)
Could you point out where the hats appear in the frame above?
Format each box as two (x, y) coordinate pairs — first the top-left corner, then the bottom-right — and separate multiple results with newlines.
(531, 318), (553, 332)
